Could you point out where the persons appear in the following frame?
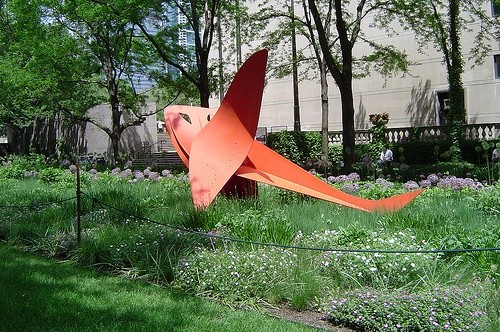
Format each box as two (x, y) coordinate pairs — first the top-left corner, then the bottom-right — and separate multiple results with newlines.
(377, 145), (394, 171)
